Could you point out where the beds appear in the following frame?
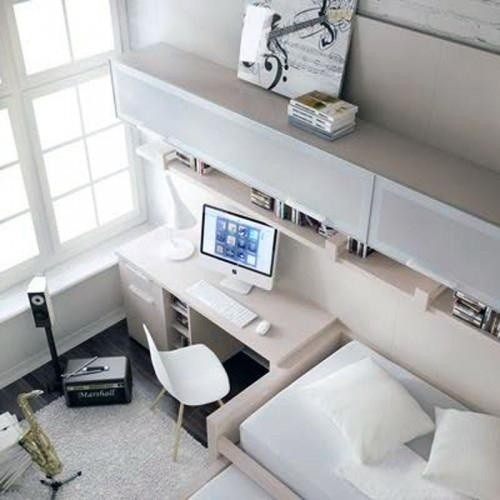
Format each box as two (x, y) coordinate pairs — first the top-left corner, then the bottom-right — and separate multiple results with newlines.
(205, 320), (500, 498)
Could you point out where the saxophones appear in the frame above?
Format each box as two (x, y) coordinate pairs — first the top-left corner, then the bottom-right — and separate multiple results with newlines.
(18, 390), (64, 477)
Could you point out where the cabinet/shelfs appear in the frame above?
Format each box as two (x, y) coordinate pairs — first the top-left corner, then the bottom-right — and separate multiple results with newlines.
(113, 223), (336, 362)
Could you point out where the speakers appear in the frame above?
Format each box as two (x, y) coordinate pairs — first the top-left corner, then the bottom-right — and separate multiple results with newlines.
(27, 277), (55, 328)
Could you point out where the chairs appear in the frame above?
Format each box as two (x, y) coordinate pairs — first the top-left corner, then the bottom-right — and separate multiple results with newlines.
(141, 323), (233, 462)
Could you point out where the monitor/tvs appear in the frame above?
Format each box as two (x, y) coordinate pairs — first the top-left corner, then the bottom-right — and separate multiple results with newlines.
(198, 203), (279, 295)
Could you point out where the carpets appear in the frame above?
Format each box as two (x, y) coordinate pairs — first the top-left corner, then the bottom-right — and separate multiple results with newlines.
(1, 382), (228, 500)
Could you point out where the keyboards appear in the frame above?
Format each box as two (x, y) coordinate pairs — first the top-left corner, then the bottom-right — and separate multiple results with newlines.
(185, 279), (258, 328)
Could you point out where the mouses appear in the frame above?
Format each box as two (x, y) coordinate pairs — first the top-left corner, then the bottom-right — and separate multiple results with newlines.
(256, 320), (270, 335)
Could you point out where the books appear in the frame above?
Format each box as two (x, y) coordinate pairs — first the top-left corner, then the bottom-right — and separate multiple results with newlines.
(172, 298), (187, 312)
(448, 290), (499, 341)
(283, 90), (360, 144)
(171, 149), (213, 179)
(0, 410), (35, 496)
(247, 188), (337, 240)
(344, 233), (377, 260)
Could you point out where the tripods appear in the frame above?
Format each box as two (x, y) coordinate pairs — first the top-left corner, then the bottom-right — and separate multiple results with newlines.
(39, 471), (82, 500)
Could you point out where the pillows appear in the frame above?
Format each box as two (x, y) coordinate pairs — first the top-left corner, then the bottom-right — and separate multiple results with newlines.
(304, 358), (499, 500)
